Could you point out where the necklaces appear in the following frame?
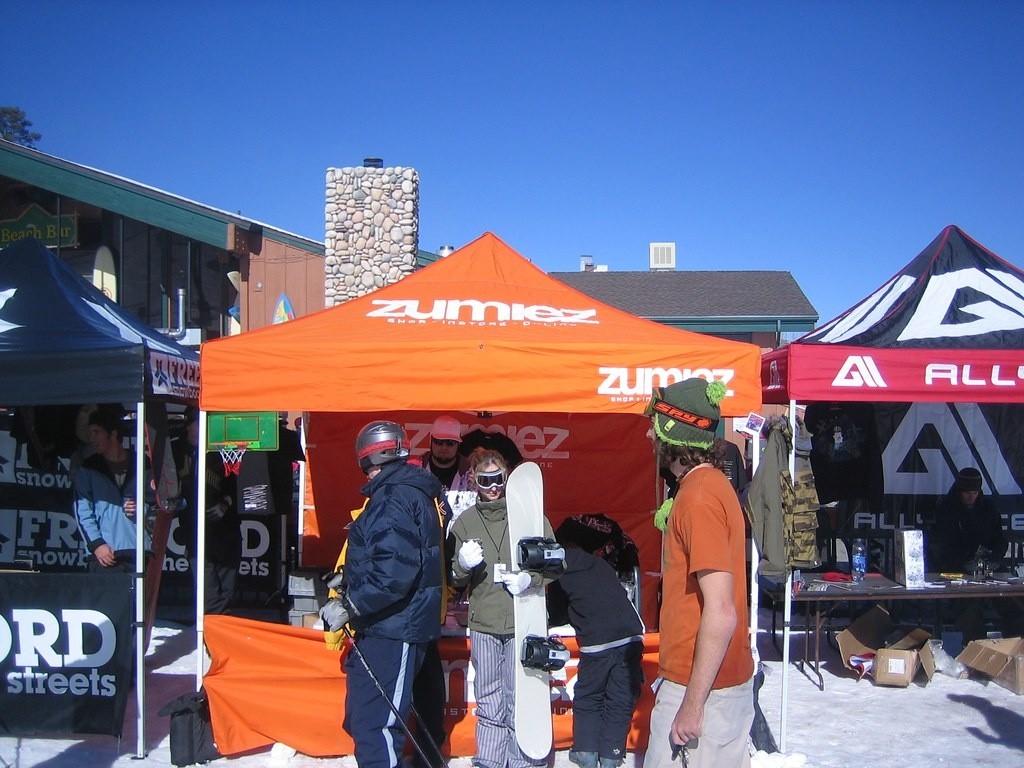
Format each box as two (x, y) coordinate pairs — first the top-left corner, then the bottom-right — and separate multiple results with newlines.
(665, 459), (714, 493)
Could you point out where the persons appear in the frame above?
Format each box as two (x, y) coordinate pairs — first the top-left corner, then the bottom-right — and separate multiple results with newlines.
(643, 377), (755, 768)
(416, 415), (468, 489)
(548, 542), (646, 768)
(928, 467), (1022, 645)
(267, 411), (307, 559)
(318, 421), (453, 768)
(73, 408), (155, 689)
(444, 449), (567, 768)
(166, 408), (243, 614)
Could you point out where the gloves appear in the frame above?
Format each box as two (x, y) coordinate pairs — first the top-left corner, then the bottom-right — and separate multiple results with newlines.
(318, 594), (361, 633)
(326, 573), (346, 594)
(458, 541), (485, 573)
(500, 571), (532, 595)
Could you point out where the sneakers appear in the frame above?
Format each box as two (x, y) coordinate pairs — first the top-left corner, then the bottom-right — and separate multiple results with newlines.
(600, 757), (624, 768)
(568, 747), (599, 768)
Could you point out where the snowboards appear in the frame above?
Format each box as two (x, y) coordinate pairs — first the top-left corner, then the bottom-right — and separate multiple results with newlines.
(504, 460), (571, 762)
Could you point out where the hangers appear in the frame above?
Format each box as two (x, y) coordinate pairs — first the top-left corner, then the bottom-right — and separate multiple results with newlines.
(783, 404), (790, 417)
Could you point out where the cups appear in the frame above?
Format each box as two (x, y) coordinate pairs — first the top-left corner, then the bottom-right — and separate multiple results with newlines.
(122, 496), (134, 513)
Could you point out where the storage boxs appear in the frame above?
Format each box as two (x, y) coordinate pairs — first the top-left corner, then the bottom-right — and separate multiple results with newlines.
(834, 602), (937, 687)
(894, 524), (924, 589)
(954, 635), (1024, 698)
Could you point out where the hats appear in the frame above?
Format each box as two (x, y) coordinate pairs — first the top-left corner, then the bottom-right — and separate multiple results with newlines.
(645, 378), (727, 450)
(957, 468), (982, 490)
(430, 415), (463, 442)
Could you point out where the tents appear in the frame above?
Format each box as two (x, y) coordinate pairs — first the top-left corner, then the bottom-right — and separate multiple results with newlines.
(1, 234), (201, 759)
(194, 230), (763, 694)
(760, 222), (1024, 754)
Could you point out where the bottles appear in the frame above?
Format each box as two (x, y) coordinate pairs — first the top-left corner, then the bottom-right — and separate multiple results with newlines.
(852, 539), (866, 582)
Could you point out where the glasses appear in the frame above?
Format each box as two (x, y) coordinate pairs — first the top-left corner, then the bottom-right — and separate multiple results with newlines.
(645, 385), (662, 415)
(475, 467), (508, 490)
(433, 438), (458, 447)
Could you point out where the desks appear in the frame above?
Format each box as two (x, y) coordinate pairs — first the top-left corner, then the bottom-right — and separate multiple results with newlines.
(758, 559), (1024, 692)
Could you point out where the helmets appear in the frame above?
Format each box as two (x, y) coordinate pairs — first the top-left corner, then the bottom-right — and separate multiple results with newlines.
(356, 420), (411, 472)
